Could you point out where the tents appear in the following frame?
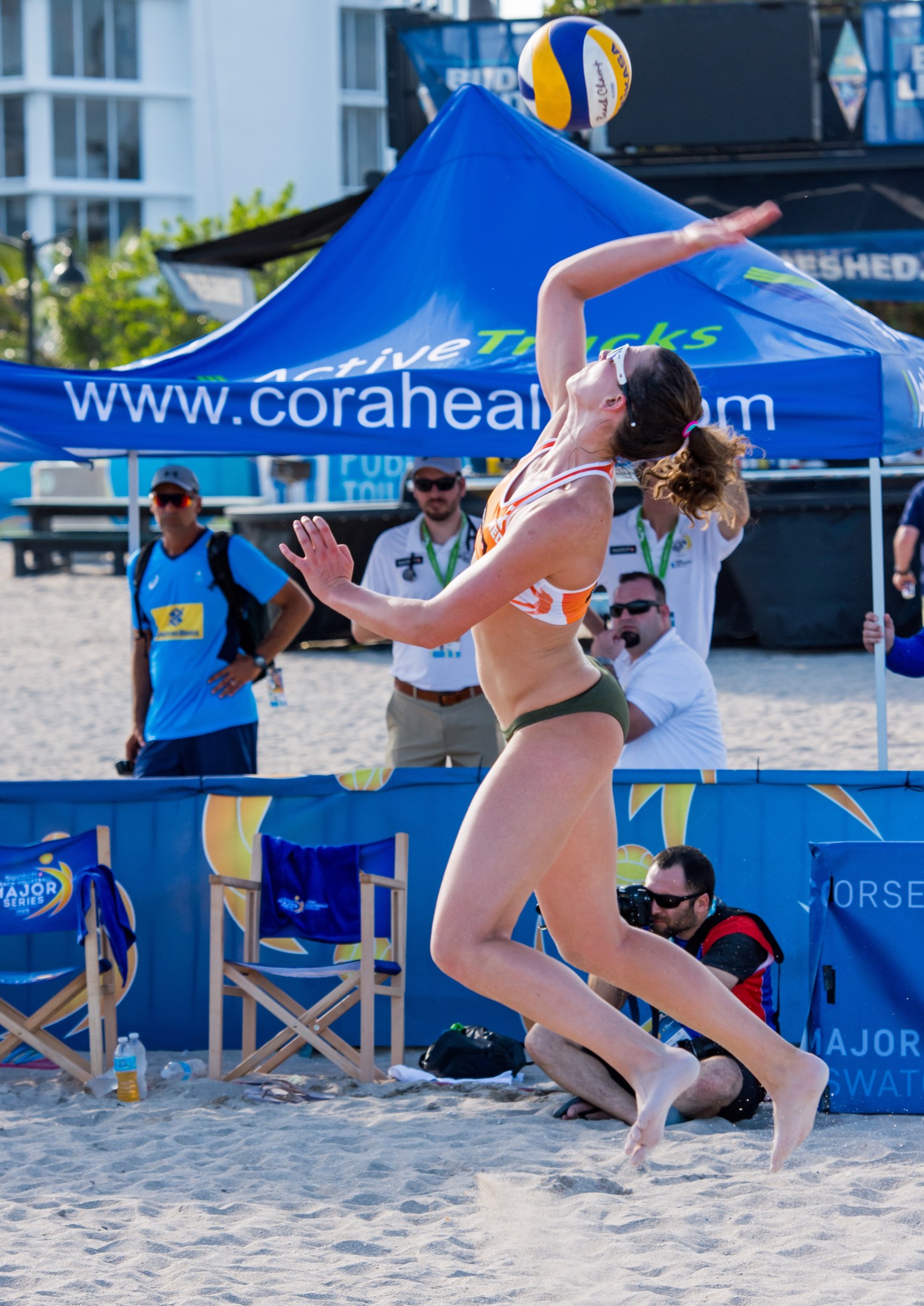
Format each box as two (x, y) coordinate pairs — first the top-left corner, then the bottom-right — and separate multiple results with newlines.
(0, 83), (924, 768)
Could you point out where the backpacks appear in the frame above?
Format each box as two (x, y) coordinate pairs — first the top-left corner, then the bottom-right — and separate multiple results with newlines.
(133, 531), (275, 684)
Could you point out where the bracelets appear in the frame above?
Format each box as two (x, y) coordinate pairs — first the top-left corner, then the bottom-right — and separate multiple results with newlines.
(894, 569), (910, 574)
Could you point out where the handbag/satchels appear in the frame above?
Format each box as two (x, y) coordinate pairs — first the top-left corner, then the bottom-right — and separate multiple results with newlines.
(419, 1023), (525, 1076)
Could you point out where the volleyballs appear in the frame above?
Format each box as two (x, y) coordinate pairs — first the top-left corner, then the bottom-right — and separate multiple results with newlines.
(518, 15), (633, 132)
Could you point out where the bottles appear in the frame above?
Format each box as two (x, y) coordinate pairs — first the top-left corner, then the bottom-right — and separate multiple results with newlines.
(128, 1032), (148, 1100)
(902, 570), (916, 599)
(159, 1058), (208, 1081)
(113, 1037), (140, 1101)
(84, 1068), (118, 1098)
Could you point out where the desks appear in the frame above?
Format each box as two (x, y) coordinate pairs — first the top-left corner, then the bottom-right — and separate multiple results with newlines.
(11, 496), (268, 576)
(226, 499), (427, 652)
(459, 464), (924, 652)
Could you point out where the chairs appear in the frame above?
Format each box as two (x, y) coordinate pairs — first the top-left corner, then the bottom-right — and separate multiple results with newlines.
(0, 824), (139, 1101)
(207, 832), (409, 1088)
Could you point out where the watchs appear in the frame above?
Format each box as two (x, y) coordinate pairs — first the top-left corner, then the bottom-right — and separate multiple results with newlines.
(251, 652), (269, 670)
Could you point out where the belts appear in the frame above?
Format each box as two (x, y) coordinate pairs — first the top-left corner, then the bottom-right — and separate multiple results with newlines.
(395, 677), (484, 707)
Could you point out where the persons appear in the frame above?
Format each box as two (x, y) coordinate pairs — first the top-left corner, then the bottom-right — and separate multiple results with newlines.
(351, 454), (506, 766)
(523, 845), (785, 1127)
(862, 612), (924, 678)
(277, 197), (833, 1170)
(590, 571), (725, 769)
(580, 459), (751, 665)
(891, 479), (924, 627)
(120, 464), (314, 779)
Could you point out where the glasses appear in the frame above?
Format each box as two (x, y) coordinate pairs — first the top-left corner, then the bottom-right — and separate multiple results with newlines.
(413, 476), (460, 492)
(608, 599), (660, 618)
(153, 494), (193, 509)
(647, 889), (701, 909)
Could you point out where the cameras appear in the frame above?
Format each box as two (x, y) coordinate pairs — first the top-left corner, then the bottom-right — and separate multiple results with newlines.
(617, 884), (652, 929)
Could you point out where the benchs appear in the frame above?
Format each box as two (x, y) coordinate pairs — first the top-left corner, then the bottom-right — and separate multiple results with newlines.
(0, 523), (165, 579)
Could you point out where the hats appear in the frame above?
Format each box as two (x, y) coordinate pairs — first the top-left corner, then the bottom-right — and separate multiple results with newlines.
(406, 456), (461, 478)
(151, 465), (197, 492)
